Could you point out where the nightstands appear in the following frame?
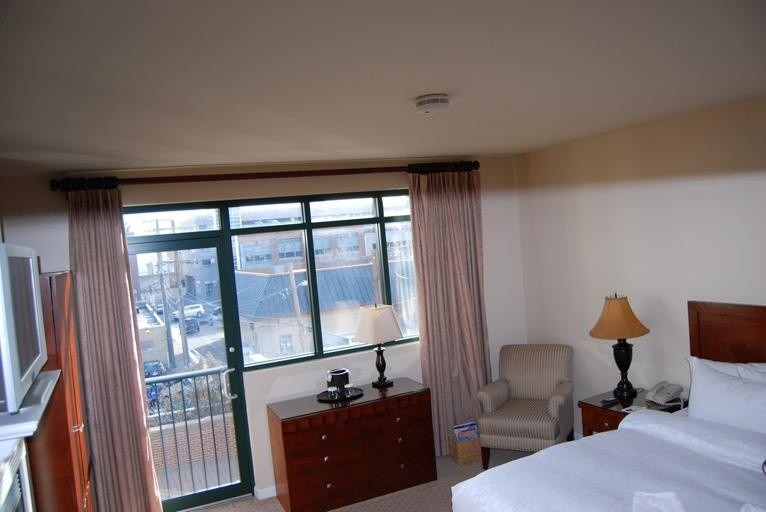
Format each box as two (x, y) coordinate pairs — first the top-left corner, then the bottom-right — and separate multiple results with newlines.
(577, 387), (687, 438)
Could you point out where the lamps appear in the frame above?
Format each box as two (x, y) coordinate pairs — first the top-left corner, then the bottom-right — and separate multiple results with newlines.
(589, 290), (650, 403)
(351, 301), (405, 389)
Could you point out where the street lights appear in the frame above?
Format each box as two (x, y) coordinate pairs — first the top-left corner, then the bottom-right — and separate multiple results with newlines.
(288, 262), (308, 351)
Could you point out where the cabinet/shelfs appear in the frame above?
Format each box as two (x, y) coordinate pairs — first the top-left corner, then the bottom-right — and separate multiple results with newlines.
(266, 375), (438, 512)
(28, 273), (98, 512)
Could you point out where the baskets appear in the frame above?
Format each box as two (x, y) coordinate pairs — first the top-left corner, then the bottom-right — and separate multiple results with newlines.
(453, 438), (482, 464)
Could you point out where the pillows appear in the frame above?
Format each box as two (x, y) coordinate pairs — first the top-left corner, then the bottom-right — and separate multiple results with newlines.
(683, 351), (765, 431)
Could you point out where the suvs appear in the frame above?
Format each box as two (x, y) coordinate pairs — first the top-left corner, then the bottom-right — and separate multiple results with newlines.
(184, 318), (200, 333)
(172, 304), (204, 319)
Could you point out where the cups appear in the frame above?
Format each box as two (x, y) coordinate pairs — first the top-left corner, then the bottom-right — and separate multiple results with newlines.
(344, 383), (354, 398)
(327, 386), (338, 400)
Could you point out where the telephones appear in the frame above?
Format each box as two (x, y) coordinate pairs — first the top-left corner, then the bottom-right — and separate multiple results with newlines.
(645, 381), (684, 405)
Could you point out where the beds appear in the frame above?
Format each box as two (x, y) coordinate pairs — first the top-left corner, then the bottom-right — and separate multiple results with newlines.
(452, 302), (765, 512)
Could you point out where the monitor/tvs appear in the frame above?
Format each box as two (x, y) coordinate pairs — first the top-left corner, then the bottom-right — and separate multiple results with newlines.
(0, 241), (52, 414)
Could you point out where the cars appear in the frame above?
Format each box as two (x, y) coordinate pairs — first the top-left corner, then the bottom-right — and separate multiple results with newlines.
(143, 360), (168, 403)
(157, 304), (164, 314)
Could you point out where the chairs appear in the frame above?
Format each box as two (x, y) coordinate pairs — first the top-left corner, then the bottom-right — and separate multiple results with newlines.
(472, 344), (574, 470)
